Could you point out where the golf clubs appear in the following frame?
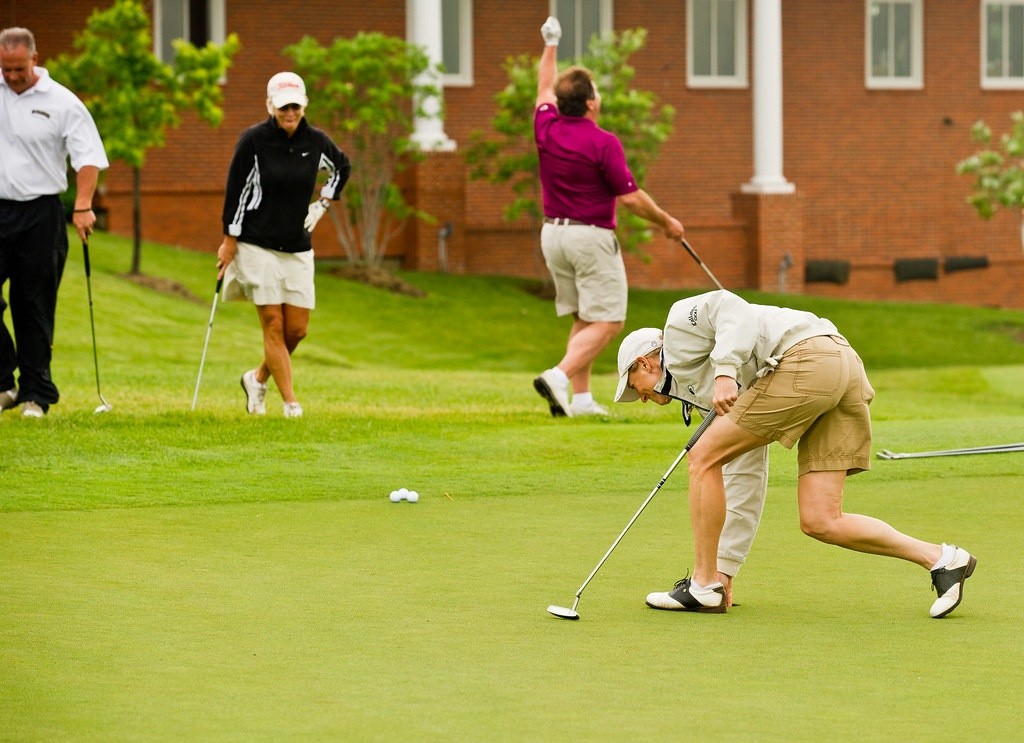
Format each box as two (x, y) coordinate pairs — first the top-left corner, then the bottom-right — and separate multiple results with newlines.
(82, 231), (113, 415)
(192, 264), (225, 410)
(876, 442), (1024, 461)
(547, 409), (716, 622)
(680, 237), (724, 290)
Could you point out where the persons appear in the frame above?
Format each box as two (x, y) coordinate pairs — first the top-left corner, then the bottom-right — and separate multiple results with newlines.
(614, 291), (978, 618)
(533, 15), (685, 417)
(217, 73), (350, 416)
(0, 25), (107, 417)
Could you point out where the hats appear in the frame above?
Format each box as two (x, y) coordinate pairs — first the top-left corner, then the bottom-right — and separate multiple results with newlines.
(266, 72), (307, 108)
(613, 328), (663, 402)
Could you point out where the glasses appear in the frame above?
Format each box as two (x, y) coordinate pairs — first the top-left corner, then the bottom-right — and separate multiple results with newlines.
(278, 103), (300, 111)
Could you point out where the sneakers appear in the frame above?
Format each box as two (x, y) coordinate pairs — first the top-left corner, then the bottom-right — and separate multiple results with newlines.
(645, 568), (727, 613)
(284, 401), (303, 418)
(929, 545), (978, 617)
(0, 388), (20, 412)
(240, 369), (270, 415)
(23, 402), (44, 418)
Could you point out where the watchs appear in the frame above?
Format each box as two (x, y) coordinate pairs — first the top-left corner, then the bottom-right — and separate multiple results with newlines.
(320, 197), (330, 211)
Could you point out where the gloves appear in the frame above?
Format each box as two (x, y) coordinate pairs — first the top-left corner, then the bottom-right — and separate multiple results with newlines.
(540, 16), (562, 46)
(304, 200), (327, 232)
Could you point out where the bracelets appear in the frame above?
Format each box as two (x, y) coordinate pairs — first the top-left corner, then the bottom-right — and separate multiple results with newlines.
(72, 208), (92, 213)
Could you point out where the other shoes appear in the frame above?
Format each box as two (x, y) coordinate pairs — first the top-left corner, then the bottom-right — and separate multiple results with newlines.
(534, 369), (571, 417)
(569, 401), (608, 416)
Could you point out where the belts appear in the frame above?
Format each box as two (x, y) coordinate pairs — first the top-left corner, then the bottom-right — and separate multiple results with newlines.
(543, 217), (588, 226)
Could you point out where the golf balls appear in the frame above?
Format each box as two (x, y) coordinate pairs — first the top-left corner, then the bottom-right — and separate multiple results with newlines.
(390, 488), (420, 503)
